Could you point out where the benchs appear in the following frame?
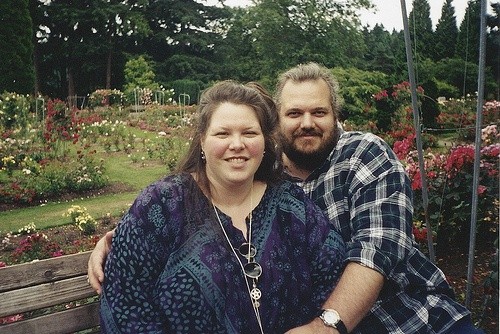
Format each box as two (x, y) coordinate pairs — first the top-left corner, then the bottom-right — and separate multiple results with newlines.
(0, 251), (100, 334)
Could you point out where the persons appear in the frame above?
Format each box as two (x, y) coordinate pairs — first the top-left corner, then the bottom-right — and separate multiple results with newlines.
(98, 82), (351, 334)
(88, 62), (492, 334)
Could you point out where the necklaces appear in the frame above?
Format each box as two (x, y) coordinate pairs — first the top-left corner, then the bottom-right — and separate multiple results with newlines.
(210, 172), (264, 334)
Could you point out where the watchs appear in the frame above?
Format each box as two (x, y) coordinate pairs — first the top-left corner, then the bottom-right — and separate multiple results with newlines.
(316, 308), (347, 334)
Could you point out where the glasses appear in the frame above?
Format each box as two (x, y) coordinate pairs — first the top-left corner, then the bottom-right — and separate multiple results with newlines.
(234, 243), (262, 287)
